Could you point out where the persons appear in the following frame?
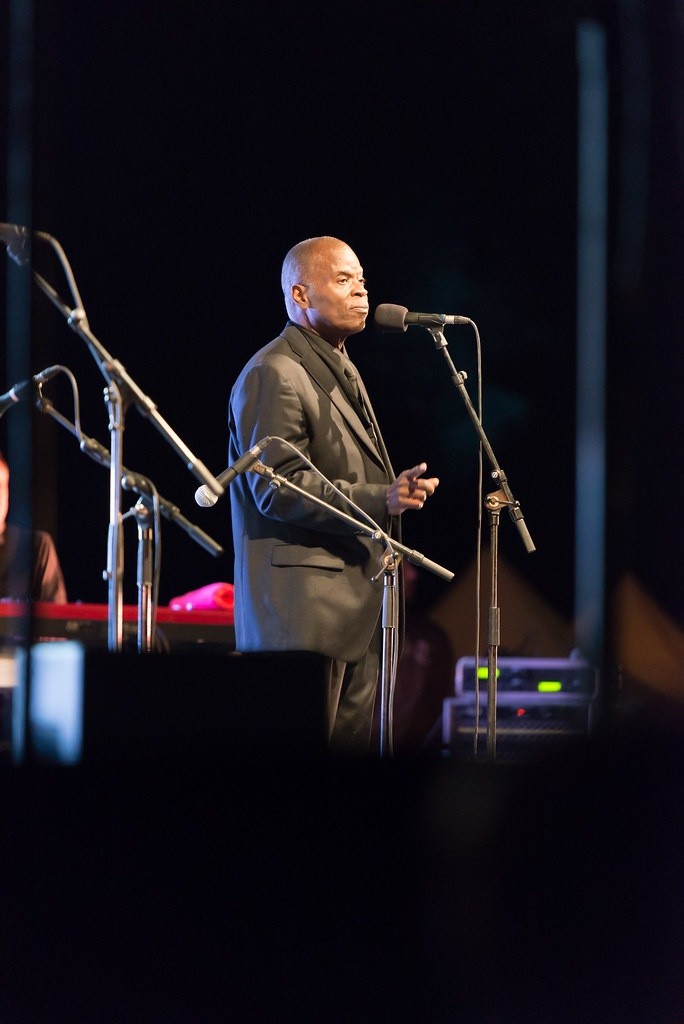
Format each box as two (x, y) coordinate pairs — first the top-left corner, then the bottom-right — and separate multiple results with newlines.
(228, 236), (439, 740)
(0, 458), (69, 640)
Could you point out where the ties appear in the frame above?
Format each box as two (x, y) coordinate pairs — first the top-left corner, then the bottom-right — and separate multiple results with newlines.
(344, 361), (359, 403)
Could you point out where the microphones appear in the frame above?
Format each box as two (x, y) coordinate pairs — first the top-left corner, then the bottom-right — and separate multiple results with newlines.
(0, 224), (51, 243)
(195, 436), (272, 508)
(0, 366), (60, 417)
(375, 304), (471, 330)
